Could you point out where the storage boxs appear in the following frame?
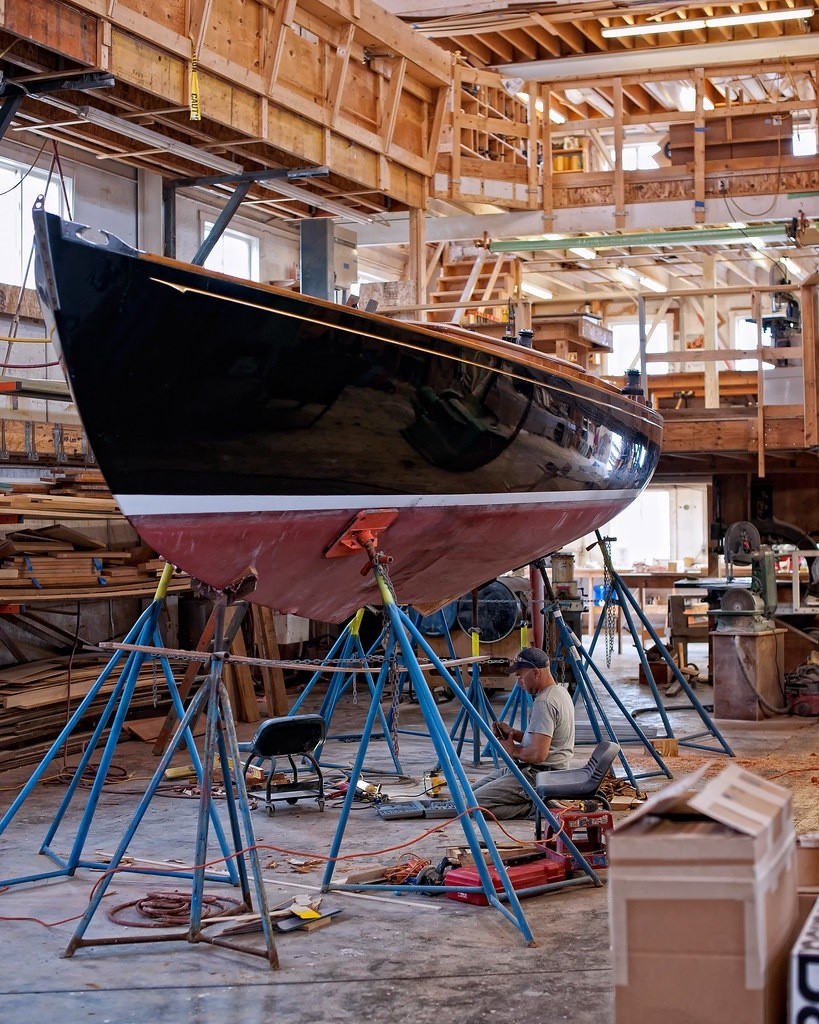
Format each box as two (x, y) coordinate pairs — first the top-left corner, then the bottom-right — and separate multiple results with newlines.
(605, 756), (799, 1024)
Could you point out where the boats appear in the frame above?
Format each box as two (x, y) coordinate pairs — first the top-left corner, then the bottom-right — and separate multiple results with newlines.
(32, 207), (666, 624)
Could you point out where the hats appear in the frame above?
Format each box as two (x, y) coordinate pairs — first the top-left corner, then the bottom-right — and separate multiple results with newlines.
(504, 648), (550, 674)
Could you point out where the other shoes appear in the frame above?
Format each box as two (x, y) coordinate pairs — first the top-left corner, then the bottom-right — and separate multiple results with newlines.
(545, 799), (568, 810)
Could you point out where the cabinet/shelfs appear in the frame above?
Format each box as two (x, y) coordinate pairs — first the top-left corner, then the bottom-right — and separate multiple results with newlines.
(708, 628), (789, 723)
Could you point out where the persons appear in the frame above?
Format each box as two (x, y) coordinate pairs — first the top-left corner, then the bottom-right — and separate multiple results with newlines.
(466, 646), (575, 820)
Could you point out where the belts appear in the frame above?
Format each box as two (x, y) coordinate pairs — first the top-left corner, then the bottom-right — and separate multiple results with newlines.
(514, 758), (565, 772)
